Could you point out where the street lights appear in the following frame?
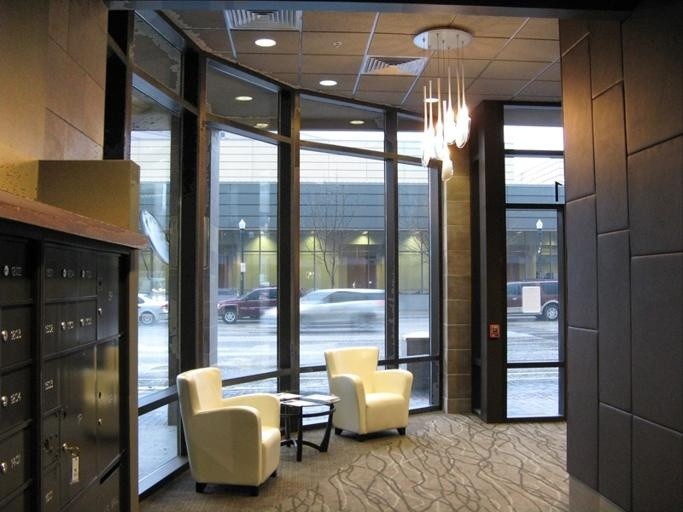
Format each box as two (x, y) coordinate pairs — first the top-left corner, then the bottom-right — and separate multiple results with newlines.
(237, 218), (245, 295)
(362, 231), (369, 288)
(414, 230), (423, 294)
(535, 218), (544, 279)
(257, 230), (265, 282)
(309, 231), (316, 290)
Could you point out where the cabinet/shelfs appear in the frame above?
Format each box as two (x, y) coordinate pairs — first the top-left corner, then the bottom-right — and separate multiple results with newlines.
(0, 216), (130, 512)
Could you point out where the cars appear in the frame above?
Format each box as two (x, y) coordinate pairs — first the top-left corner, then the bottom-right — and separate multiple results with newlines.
(137, 293), (168, 324)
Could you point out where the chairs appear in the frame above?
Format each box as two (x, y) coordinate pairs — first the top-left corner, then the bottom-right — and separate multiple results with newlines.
(176, 366), (282, 497)
(324, 346), (414, 442)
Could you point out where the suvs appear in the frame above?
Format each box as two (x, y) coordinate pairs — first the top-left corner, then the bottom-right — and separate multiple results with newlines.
(506, 279), (558, 322)
(216, 286), (307, 323)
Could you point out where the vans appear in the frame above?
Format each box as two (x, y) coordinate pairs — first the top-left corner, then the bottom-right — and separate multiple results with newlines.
(262, 289), (385, 331)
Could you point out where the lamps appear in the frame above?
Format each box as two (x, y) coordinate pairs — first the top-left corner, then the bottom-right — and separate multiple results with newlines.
(413, 25), (474, 184)
